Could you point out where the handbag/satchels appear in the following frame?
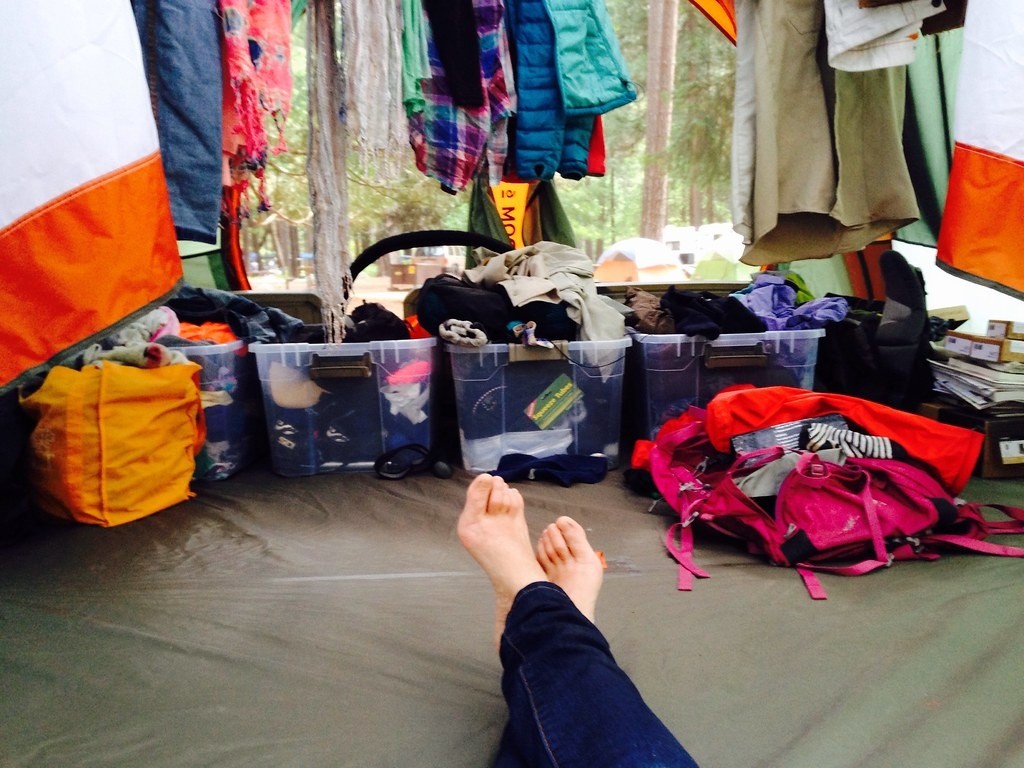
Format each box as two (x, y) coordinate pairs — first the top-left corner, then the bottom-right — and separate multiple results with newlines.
(17, 360), (208, 528)
(646, 411), (1024, 597)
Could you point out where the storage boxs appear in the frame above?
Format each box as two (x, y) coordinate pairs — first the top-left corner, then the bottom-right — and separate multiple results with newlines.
(945, 319), (1024, 363)
(918, 402), (1024, 479)
(168, 339), (257, 481)
(442, 330), (633, 475)
(628, 326), (826, 441)
(249, 337), (440, 477)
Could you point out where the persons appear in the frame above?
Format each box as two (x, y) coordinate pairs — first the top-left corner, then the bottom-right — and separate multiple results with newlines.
(455, 474), (700, 768)
